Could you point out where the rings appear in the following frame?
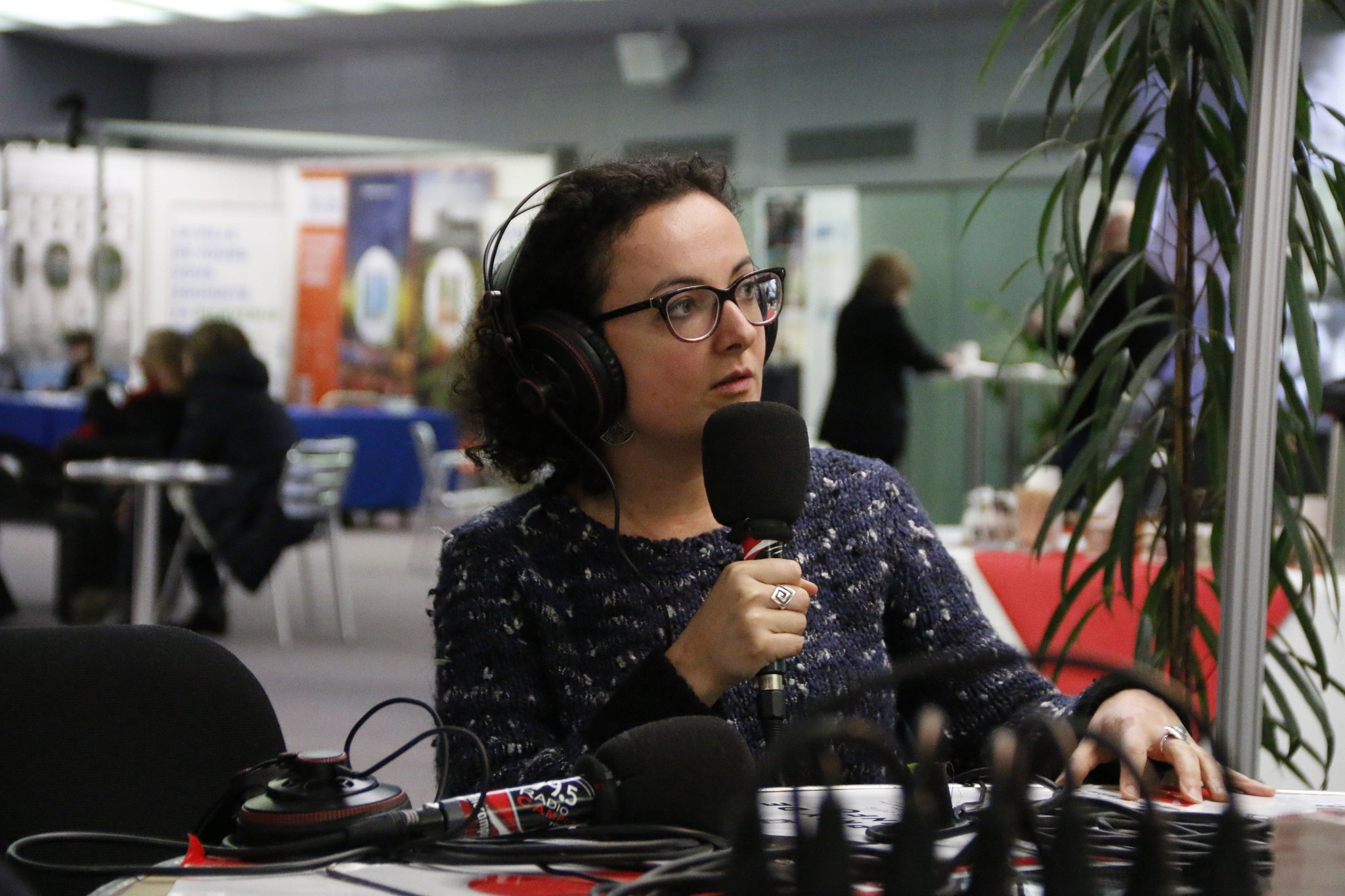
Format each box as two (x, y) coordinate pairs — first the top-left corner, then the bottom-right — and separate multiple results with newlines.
(770, 584), (796, 610)
(1159, 725), (1190, 753)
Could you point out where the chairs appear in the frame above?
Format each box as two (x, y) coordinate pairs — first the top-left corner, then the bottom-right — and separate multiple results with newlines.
(272, 437), (355, 647)
(0, 624), (287, 896)
(411, 424), (511, 579)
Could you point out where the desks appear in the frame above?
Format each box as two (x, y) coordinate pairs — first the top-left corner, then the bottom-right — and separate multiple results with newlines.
(87, 785), (1345, 895)
(948, 361), (1074, 493)
(64, 459), (231, 623)
(0, 388), (463, 531)
(936, 523), (1345, 794)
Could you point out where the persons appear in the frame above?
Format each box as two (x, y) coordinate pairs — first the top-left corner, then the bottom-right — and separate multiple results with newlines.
(1024, 196), (1176, 533)
(43, 323), (319, 637)
(58, 328), (99, 390)
(816, 251), (951, 469)
(426, 153), (1278, 845)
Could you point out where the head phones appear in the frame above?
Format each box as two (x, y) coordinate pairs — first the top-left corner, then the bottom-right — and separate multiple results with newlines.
(232, 751), (411, 852)
(472, 170), (779, 437)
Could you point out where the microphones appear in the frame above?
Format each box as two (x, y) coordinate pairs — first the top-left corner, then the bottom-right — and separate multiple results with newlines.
(700, 399), (810, 722)
(345, 712), (757, 844)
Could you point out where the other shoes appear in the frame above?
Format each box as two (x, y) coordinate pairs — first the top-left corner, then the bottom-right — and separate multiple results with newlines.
(172, 606), (228, 638)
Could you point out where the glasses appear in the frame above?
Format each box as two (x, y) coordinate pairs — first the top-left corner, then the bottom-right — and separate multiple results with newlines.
(597, 267), (787, 343)
